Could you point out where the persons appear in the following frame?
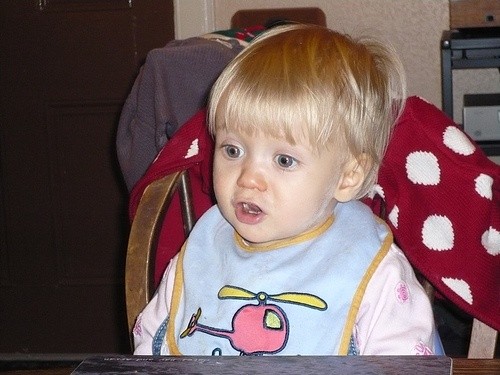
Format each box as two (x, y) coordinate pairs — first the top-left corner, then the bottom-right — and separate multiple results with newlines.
(131, 21), (449, 355)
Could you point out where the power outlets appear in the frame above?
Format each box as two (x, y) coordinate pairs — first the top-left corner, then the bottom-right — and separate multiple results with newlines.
(485, 13), (496, 24)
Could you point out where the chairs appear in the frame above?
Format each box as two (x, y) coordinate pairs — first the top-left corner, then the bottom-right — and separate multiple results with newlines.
(125, 96), (500, 355)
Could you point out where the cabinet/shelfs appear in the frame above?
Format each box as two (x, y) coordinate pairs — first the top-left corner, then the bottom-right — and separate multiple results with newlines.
(440, 30), (500, 157)
